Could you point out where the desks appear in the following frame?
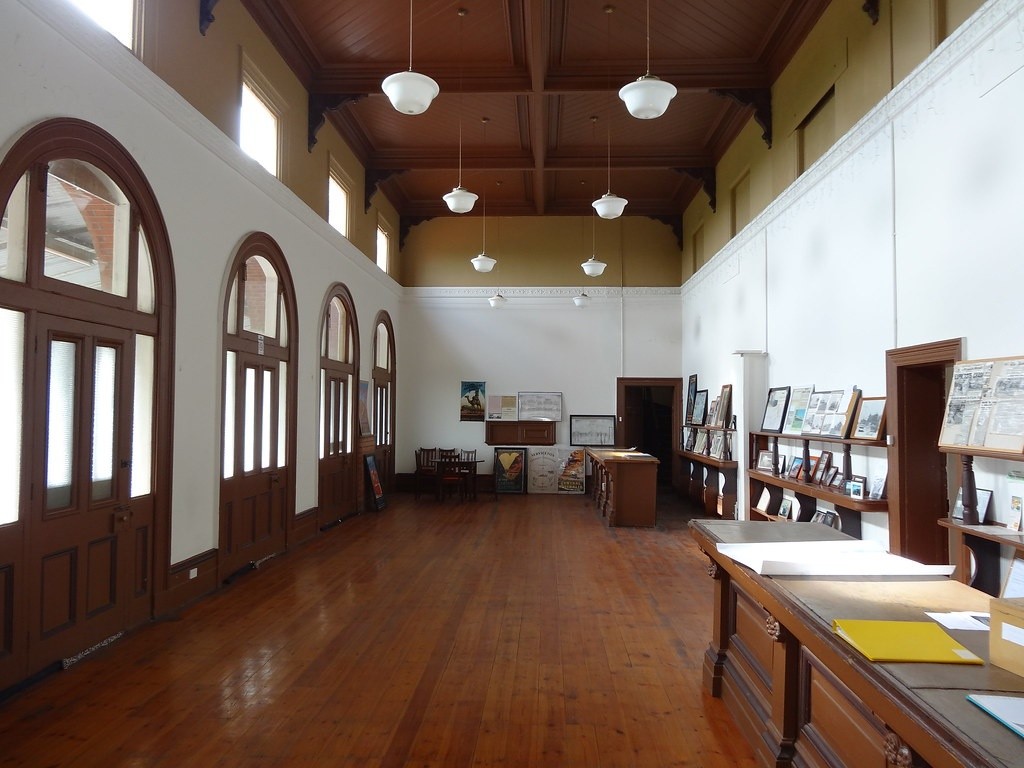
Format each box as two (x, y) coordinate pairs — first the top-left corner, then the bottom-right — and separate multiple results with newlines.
(685, 517), (1024, 767)
(585, 448), (659, 528)
(429, 459), (485, 503)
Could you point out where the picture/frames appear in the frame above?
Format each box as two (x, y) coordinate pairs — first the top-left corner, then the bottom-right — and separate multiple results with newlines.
(685, 429), (733, 459)
(759, 385), (886, 440)
(952, 486), (993, 524)
(366, 453), (387, 513)
(683, 374), (736, 428)
(810, 511), (837, 527)
(756, 449), (886, 499)
(494, 447), (528, 495)
(778, 498), (791, 519)
(570, 414), (616, 447)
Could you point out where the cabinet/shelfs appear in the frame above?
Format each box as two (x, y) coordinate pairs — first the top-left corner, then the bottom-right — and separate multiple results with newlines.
(675, 425), (737, 520)
(748, 432), (888, 523)
(938, 445), (1024, 603)
(485, 420), (556, 447)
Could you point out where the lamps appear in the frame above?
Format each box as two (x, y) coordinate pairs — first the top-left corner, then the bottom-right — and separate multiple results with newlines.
(380, 2), (439, 115)
(592, 122), (628, 221)
(470, 115), (497, 272)
(488, 181), (509, 308)
(618, 1), (678, 122)
(444, 8), (479, 214)
(583, 111), (607, 276)
(573, 179), (596, 309)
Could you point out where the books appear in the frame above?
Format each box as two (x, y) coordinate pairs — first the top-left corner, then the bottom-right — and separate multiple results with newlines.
(965, 695), (1024, 740)
(833, 618), (985, 665)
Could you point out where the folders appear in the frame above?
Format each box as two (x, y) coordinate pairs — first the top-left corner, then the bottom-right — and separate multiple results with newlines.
(832, 619), (986, 665)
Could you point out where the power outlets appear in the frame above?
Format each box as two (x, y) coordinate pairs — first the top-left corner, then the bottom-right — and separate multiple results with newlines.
(189, 568), (197, 579)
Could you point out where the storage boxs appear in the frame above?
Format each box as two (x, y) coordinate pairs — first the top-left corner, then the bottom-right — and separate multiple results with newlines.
(987, 597), (1024, 676)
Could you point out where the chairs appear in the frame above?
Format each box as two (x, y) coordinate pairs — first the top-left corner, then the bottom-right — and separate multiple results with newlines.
(415, 447), (477, 504)
